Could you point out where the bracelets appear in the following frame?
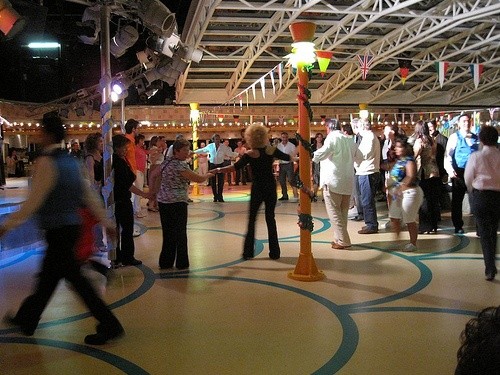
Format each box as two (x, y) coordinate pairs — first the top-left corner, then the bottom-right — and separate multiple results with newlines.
(217, 168), (221, 173)
(295, 157), (299, 161)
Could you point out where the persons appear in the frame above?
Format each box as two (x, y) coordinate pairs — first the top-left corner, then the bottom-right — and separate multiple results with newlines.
(454, 304), (500, 375)
(8, 114), (500, 283)
(0, 113), (125, 346)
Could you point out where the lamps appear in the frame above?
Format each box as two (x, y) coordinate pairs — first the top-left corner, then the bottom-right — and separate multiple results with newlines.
(0, 0), (194, 118)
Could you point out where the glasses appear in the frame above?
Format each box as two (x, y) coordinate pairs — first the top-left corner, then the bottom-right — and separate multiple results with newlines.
(162, 140), (166, 144)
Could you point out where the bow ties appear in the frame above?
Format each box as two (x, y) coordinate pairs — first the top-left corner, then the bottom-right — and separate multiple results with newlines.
(465, 133), (472, 138)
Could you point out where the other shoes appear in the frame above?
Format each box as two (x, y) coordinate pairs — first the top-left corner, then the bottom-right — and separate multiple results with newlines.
(430, 225), (438, 232)
(242, 253), (254, 260)
(269, 251), (280, 260)
(123, 259), (141, 266)
(418, 228), (432, 234)
(8, 316), (37, 336)
(147, 208), (158, 212)
(218, 199), (223, 202)
(454, 227), (464, 234)
(214, 197), (217, 202)
(402, 243), (417, 252)
(353, 216), (365, 221)
(85, 326), (125, 344)
(133, 229), (140, 236)
(486, 269), (496, 282)
(279, 195), (288, 201)
(358, 226), (379, 234)
(188, 199), (193, 202)
(476, 234), (480, 238)
(330, 241), (351, 249)
(385, 220), (407, 230)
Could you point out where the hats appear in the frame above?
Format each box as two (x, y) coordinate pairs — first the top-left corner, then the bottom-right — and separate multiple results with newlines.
(35, 112), (64, 126)
